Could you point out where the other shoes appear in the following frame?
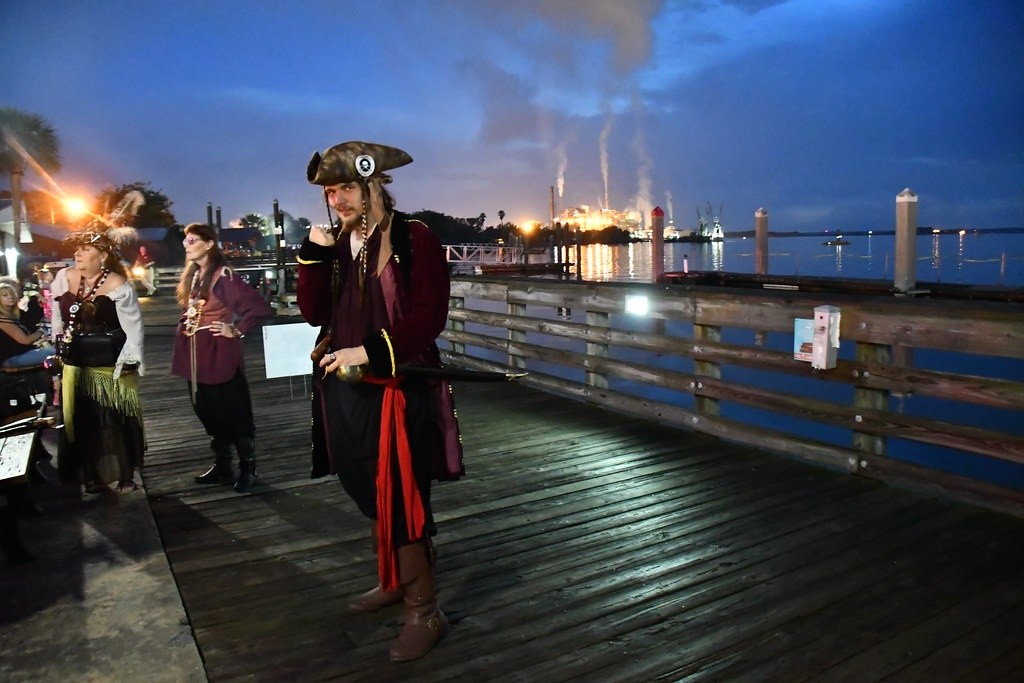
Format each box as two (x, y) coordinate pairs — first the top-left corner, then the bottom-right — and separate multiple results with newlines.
(115, 479), (140, 495)
(53, 397), (59, 405)
(86, 485), (106, 493)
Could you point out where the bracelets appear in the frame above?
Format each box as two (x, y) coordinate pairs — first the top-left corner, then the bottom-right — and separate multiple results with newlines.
(231, 328), (243, 339)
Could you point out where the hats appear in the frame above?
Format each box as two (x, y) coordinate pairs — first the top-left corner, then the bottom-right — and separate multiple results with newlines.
(307, 141), (413, 186)
(62, 191), (146, 262)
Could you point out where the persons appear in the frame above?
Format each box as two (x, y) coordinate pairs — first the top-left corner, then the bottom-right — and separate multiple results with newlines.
(296, 140), (451, 662)
(168, 223), (275, 493)
(49, 232), (145, 501)
(132, 246), (157, 297)
(0, 266), (61, 407)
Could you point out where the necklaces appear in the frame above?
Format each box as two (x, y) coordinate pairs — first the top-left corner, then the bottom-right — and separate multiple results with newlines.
(184, 270), (202, 332)
(84, 280), (95, 293)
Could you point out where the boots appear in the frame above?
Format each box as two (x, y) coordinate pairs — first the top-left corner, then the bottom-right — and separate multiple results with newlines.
(233, 457), (257, 492)
(348, 519), (406, 612)
(193, 444), (234, 483)
(388, 540), (448, 662)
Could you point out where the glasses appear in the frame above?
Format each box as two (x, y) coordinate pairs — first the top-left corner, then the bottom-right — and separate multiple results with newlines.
(182, 237), (203, 248)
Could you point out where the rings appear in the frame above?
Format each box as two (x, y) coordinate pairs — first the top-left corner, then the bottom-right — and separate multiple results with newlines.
(219, 325), (222, 329)
(329, 353), (336, 360)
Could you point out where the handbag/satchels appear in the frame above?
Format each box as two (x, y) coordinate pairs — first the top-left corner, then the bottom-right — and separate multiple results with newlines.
(61, 330), (140, 370)
